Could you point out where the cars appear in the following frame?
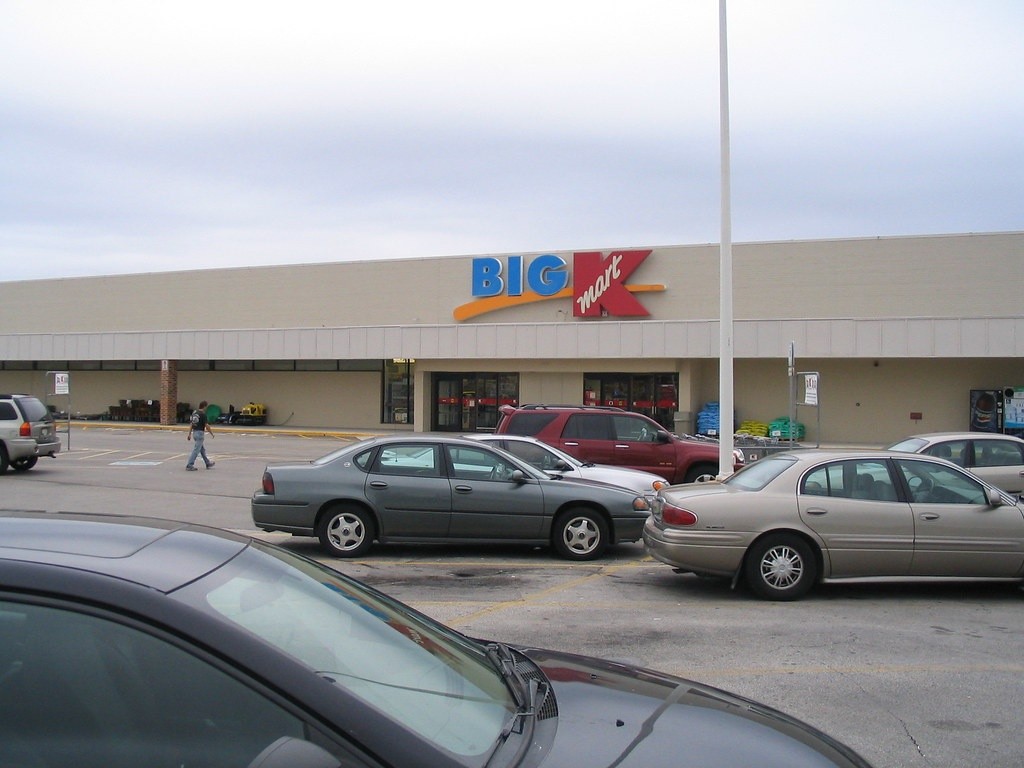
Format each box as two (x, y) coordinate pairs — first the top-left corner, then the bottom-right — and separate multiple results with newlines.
(381, 432), (671, 513)
(642, 452), (1024, 600)
(251, 432), (652, 559)
(882, 432), (1024, 495)
(0, 507), (878, 768)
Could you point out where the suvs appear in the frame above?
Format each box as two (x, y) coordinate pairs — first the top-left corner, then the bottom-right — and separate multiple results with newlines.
(1, 392), (61, 476)
(493, 402), (745, 484)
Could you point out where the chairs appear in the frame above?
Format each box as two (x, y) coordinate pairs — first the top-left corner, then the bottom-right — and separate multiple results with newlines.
(937, 445), (952, 457)
(851, 474), (874, 499)
(868, 480), (893, 501)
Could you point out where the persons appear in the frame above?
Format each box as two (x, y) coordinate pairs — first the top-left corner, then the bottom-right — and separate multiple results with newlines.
(185, 401), (216, 471)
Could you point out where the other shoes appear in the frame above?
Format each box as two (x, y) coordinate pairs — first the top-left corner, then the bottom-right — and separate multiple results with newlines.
(186, 466), (198, 471)
(206, 462), (215, 469)
